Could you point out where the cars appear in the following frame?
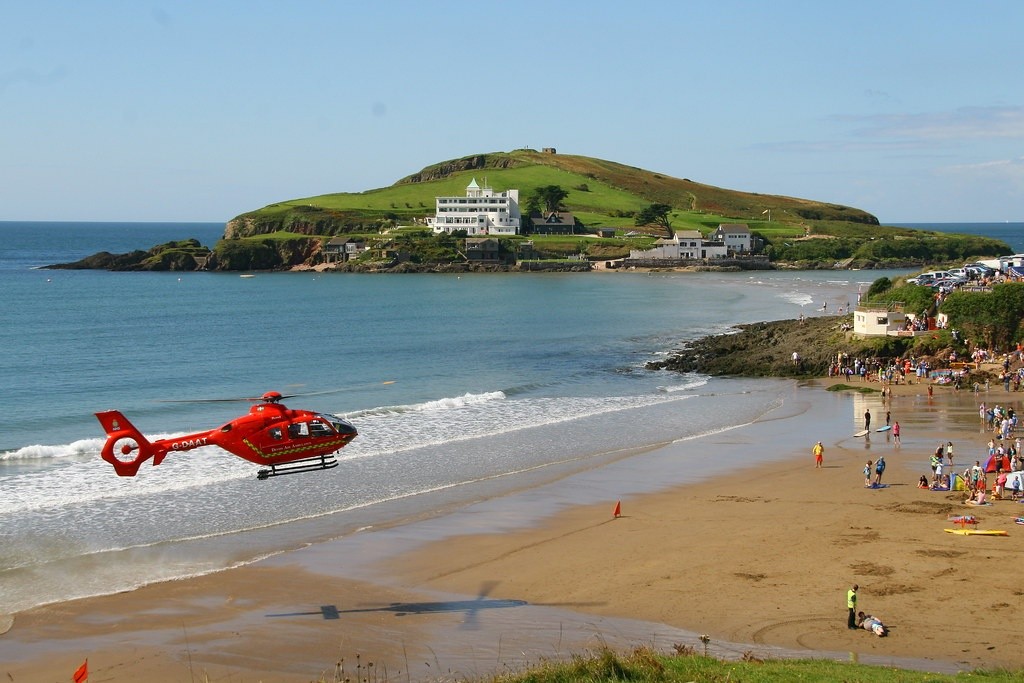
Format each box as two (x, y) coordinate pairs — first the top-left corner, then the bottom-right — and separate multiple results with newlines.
(906, 263), (998, 291)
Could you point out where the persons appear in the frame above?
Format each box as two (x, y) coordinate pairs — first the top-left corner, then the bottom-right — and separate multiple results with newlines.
(857, 611), (885, 637)
(789, 269), (1024, 400)
(865, 409), (871, 430)
(813, 442), (824, 468)
(886, 411), (891, 427)
(863, 460), (872, 486)
(930, 401), (1024, 507)
(894, 422), (900, 444)
(916, 475), (928, 488)
(874, 456), (885, 485)
(847, 584), (858, 629)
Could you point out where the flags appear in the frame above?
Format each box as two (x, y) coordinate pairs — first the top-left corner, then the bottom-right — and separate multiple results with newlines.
(72, 658), (88, 683)
(614, 501), (620, 518)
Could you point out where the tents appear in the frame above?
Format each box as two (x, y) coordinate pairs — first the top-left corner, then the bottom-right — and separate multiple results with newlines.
(982, 453), (1012, 473)
(1005, 470), (1024, 492)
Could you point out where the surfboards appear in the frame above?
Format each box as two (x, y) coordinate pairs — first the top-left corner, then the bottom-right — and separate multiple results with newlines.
(875, 425), (891, 431)
(853, 430), (868, 437)
(943, 527), (1006, 535)
(888, 367), (918, 370)
(948, 363), (981, 369)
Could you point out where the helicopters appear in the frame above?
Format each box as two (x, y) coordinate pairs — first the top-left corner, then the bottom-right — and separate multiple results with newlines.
(94, 379), (399, 480)
